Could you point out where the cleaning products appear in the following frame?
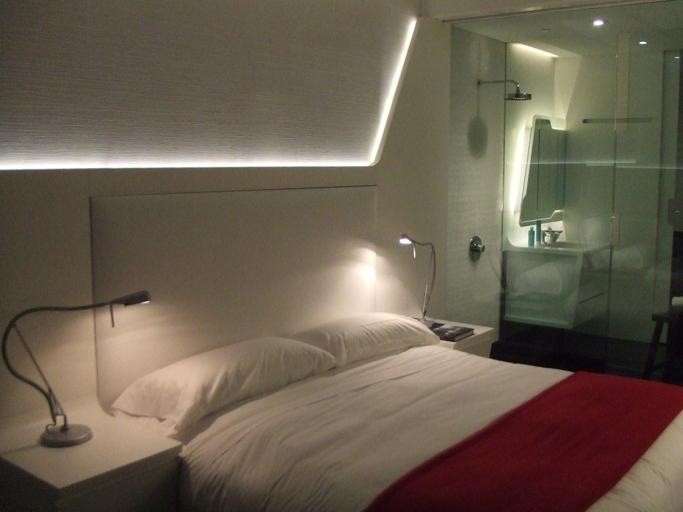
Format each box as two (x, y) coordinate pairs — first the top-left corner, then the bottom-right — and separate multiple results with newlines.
(536, 219), (541, 246)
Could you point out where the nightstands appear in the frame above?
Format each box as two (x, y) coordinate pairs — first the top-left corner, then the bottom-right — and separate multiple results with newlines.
(0, 410), (182, 512)
(426, 318), (498, 358)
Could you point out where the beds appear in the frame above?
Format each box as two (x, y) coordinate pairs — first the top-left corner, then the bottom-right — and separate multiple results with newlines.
(90, 184), (683, 512)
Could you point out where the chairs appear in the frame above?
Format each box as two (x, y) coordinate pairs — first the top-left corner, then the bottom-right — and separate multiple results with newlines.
(641, 231), (683, 383)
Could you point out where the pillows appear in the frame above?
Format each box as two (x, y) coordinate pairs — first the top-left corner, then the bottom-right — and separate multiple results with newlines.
(111, 336), (336, 437)
(290, 313), (440, 368)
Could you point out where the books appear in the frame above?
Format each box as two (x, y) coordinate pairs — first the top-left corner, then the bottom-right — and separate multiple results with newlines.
(416, 316), (475, 343)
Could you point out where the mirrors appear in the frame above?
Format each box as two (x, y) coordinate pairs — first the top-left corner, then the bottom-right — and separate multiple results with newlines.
(518, 114), (568, 226)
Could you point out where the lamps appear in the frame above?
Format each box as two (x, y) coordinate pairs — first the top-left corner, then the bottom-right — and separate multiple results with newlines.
(1, 291), (151, 448)
(399, 235), (437, 329)
